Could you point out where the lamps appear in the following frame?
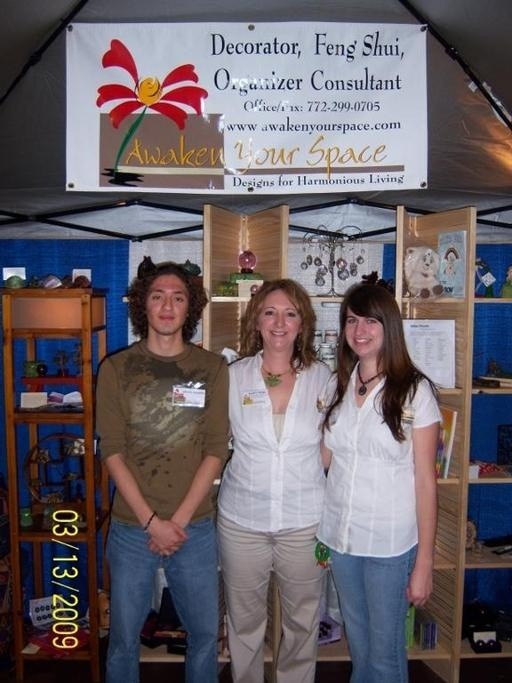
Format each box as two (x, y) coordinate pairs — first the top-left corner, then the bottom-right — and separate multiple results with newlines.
(300, 222), (367, 299)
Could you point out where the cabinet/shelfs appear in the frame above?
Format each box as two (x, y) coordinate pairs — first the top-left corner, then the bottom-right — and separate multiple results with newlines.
(0, 281), (109, 682)
(139, 198), (511, 683)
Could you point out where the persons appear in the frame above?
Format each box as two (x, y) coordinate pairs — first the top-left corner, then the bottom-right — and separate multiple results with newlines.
(320, 283), (443, 682)
(94, 262), (230, 682)
(215, 278), (338, 682)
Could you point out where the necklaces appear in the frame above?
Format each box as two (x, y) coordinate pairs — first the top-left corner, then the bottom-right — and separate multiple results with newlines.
(260, 362), (292, 385)
(357, 359), (385, 395)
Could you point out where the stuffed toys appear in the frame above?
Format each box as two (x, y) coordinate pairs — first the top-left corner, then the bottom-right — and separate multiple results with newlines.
(405, 246), (444, 299)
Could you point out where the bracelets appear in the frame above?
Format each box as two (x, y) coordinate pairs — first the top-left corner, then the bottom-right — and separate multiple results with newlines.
(144, 510), (155, 530)
